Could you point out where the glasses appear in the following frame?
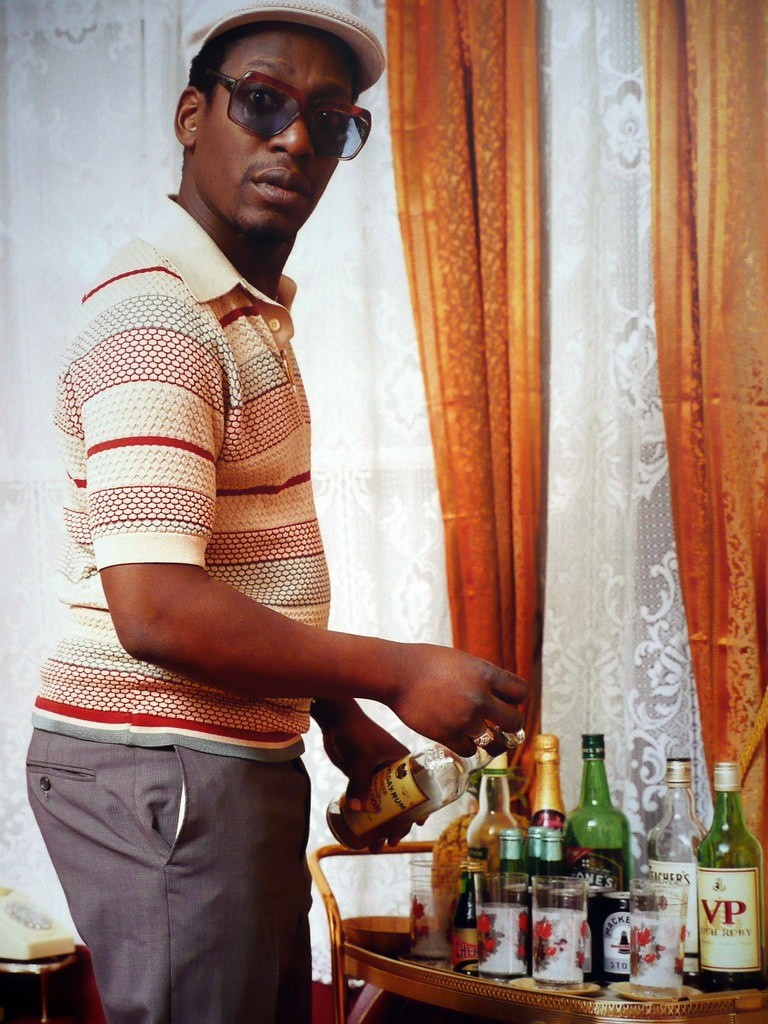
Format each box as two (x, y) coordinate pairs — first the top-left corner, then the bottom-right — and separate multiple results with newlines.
(203, 68), (371, 162)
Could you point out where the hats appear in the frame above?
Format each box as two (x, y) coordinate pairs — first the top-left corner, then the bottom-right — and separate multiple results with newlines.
(181, 0), (385, 95)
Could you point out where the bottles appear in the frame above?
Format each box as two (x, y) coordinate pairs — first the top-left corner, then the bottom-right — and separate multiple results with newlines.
(467, 750), (517, 875)
(647, 757), (707, 984)
(697, 763), (764, 992)
(453, 862), (484, 977)
(326, 725), (526, 849)
(531, 735), (567, 828)
(527, 826), (543, 904)
(459, 861), (469, 898)
(630, 878), (688, 996)
(499, 830), (526, 905)
(543, 828), (564, 907)
(564, 734), (627, 987)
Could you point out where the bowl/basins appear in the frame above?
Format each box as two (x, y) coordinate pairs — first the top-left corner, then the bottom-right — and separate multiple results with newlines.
(343, 916), (411, 953)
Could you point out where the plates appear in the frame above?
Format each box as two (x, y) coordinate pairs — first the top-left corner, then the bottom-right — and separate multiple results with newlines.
(509, 978), (600, 995)
(607, 982), (703, 1001)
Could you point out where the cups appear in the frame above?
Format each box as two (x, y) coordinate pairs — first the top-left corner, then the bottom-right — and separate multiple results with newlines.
(483, 873), (529, 984)
(409, 859), (456, 958)
(532, 877), (587, 991)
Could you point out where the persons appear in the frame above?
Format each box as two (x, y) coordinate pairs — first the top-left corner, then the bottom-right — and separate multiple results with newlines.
(24, 0), (530, 1023)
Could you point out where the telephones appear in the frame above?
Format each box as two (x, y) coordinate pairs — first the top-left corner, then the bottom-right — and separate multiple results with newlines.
(1, 888), (77, 962)
